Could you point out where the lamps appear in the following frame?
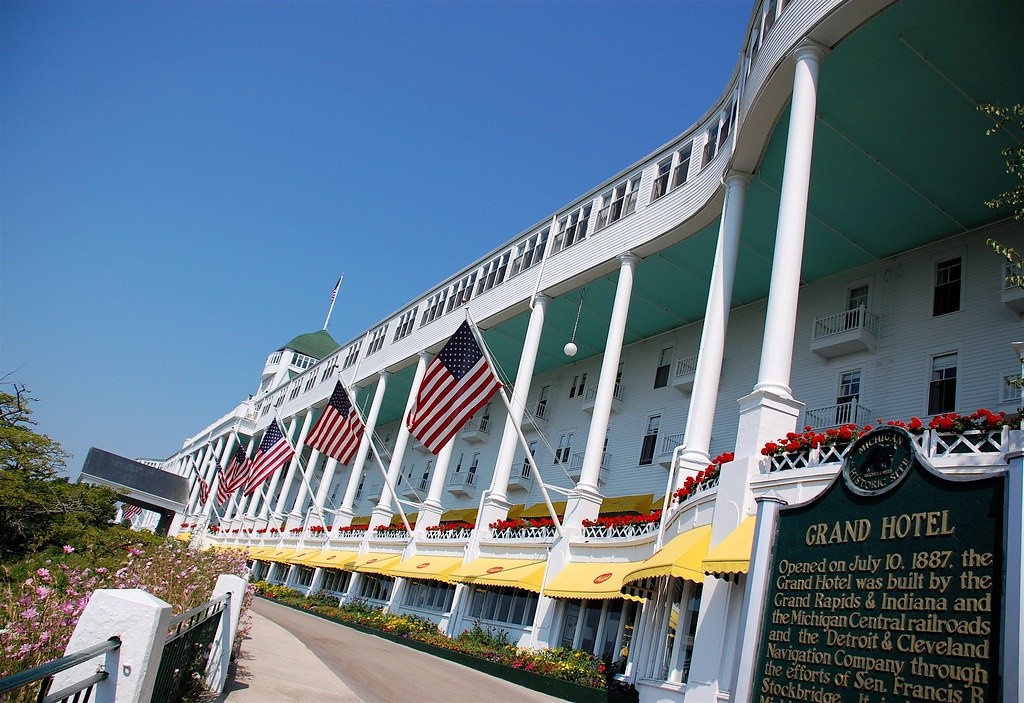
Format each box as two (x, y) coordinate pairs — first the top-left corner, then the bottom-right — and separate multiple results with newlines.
(563, 287), (585, 357)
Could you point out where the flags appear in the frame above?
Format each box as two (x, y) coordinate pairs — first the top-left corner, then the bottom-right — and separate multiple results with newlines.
(122, 503), (142, 525)
(332, 277), (342, 304)
(303, 380), (366, 468)
(192, 461), (211, 507)
(214, 452), (236, 508)
(406, 319), (505, 455)
(243, 416), (296, 497)
(224, 444), (253, 494)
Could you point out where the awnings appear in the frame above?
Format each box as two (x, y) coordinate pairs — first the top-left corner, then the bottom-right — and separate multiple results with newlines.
(700, 515), (757, 587)
(350, 515), (371, 526)
(391, 513), (418, 525)
(619, 524), (713, 633)
(447, 557), (548, 600)
(508, 501), (567, 520)
(440, 508), (479, 526)
(542, 561), (645, 613)
(599, 491), (672, 515)
(201, 543), (464, 590)
(176, 532), (191, 543)
